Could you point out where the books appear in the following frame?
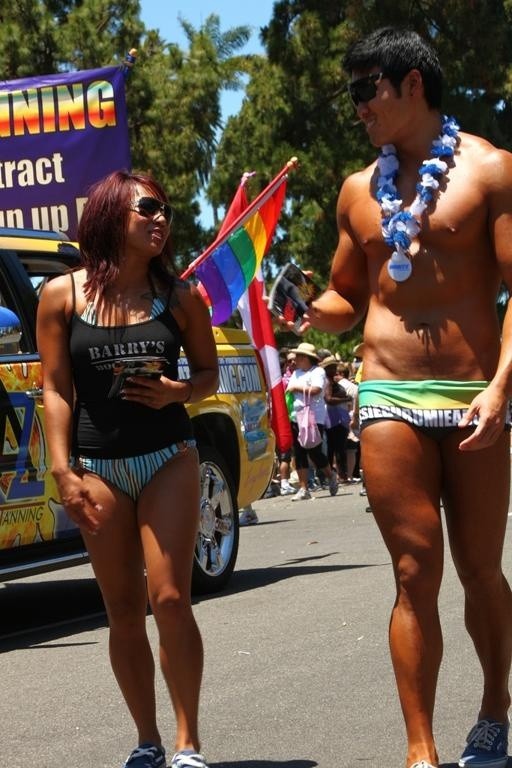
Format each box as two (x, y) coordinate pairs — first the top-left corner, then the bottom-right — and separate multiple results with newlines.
(266, 261), (324, 336)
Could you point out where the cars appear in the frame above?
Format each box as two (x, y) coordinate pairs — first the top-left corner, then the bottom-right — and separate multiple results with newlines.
(0, 226), (277, 593)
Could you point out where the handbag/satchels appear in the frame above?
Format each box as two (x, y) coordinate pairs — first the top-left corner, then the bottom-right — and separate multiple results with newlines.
(297, 386), (323, 449)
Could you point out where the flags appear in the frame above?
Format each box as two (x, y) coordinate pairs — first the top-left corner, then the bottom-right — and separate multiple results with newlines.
(196, 173), (285, 328)
(197, 180), (293, 453)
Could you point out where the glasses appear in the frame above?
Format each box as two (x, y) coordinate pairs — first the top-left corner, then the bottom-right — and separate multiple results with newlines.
(347, 73), (386, 104)
(132, 198), (174, 224)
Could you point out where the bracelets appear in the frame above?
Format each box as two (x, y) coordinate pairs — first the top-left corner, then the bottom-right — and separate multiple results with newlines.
(178, 378), (194, 404)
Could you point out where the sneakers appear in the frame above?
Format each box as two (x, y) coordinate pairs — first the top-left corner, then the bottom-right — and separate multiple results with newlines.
(173, 751), (205, 768)
(126, 746), (164, 766)
(327, 471), (367, 496)
(458, 717), (509, 767)
(264, 479), (328, 500)
(238, 509), (257, 525)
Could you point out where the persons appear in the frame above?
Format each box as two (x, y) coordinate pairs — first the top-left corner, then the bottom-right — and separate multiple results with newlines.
(37, 170), (220, 768)
(285, 26), (512, 767)
(236, 343), (374, 526)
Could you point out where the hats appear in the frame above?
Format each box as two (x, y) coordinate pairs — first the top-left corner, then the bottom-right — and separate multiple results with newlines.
(288, 342), (363, 370)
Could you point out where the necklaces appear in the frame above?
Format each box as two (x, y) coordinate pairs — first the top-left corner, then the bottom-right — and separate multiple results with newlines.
(372, 111), (461, 283)
